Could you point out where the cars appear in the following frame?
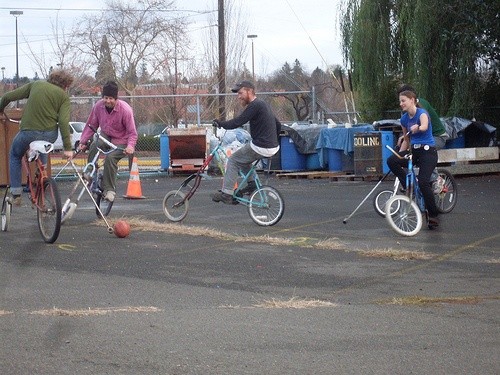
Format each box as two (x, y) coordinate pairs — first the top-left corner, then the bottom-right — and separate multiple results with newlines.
(51, 121), (92, 153)
(136, 122), (168, 139)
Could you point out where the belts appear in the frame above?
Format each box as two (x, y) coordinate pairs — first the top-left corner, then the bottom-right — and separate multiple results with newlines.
(411, 144), (435, 151)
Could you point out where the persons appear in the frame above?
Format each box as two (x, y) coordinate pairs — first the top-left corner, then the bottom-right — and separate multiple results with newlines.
(386, 90), (441, 228)
(77, 81), (139, 201)
(396, 86), (448, 195)
(210, 81), (281, 205)
(0, 70), (73, 203)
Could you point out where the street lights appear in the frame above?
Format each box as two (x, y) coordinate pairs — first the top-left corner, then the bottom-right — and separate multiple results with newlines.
(1, 67), (5, 79)
(246, 35), (258, 82)
(9, 11), (23, 107)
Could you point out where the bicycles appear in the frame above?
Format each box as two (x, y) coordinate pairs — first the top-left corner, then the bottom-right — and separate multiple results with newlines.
(374, 145), (457, 236)
(162, 120), (285, 228)
(0, 110), (61, 243)
(59, 123), (126, 226)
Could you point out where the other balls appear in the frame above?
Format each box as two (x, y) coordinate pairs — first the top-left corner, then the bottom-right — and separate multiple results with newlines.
(114, 221), (130, 238)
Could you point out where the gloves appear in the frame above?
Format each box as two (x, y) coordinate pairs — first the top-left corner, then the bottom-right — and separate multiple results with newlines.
(212, 118), (222, 129)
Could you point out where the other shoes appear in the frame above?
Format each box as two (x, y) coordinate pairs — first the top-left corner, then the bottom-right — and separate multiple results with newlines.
(210, 191), (233, 205)
(400, 186), (408, 192)
(82, 170), (94, 180)
(105, 191), (115, 202)
(246, 179), (262, 195)
(426, 209), (441, 228)
(5, 195), (22, 206)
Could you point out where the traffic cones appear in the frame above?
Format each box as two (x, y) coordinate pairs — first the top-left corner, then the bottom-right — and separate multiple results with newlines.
(122, 157), (148, 199)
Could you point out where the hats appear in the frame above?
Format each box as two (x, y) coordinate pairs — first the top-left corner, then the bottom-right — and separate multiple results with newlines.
(231, 80), (256, 93)
(102, 81), (118, 98)
